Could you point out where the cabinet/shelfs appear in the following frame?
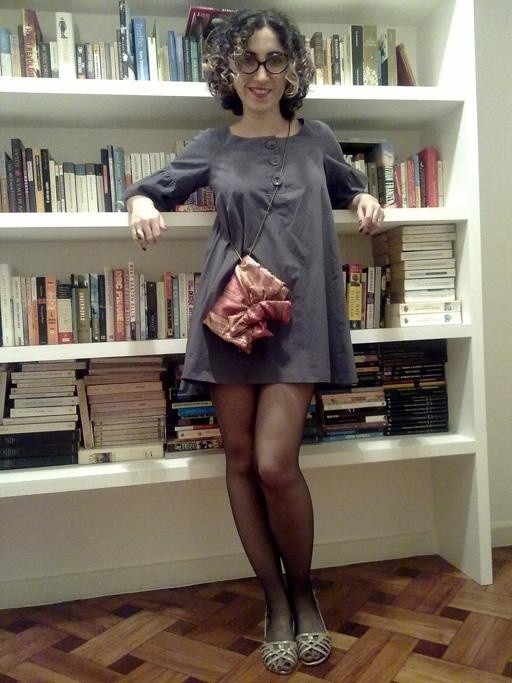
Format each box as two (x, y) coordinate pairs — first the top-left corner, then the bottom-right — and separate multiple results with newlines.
(0, 0), (492, 612)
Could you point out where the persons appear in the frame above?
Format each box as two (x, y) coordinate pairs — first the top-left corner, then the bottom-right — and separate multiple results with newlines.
(120, 6), (386, 674)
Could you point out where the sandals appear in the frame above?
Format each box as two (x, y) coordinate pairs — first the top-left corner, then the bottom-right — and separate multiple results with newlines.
(261, 604), (298, 674)
(293, 591), (332, 666)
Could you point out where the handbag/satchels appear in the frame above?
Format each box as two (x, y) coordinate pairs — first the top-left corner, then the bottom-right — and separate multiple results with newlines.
(204, 255), (292, 353)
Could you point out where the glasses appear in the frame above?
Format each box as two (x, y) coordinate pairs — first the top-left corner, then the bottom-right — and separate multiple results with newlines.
(227, 54), (288, 74)
